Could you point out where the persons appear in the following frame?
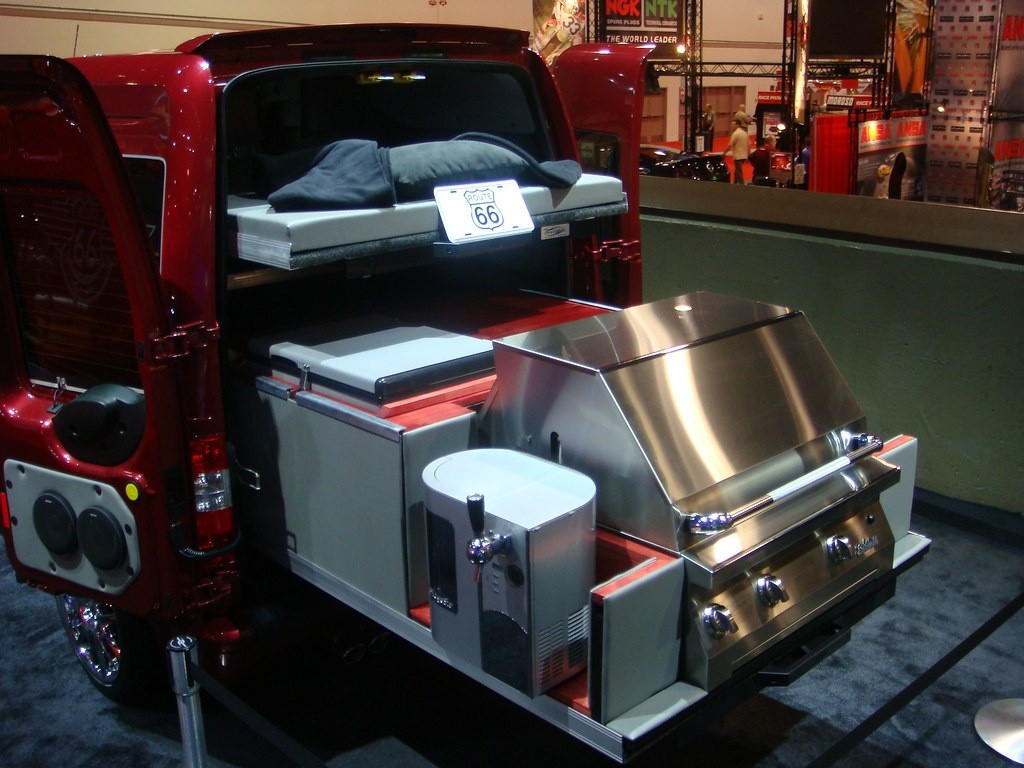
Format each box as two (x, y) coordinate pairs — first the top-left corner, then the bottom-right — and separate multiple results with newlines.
(702, 103), (716, 152)
(734, 101), (759, 132)
(721, 119), (811, 190)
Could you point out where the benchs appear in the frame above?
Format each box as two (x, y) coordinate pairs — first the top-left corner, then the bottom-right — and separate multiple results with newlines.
(213, 60), (633, 294)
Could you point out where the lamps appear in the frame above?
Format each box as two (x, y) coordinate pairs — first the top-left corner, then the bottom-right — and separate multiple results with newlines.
(931, 99), (949, 112)
(882, 109), (892, 120)
